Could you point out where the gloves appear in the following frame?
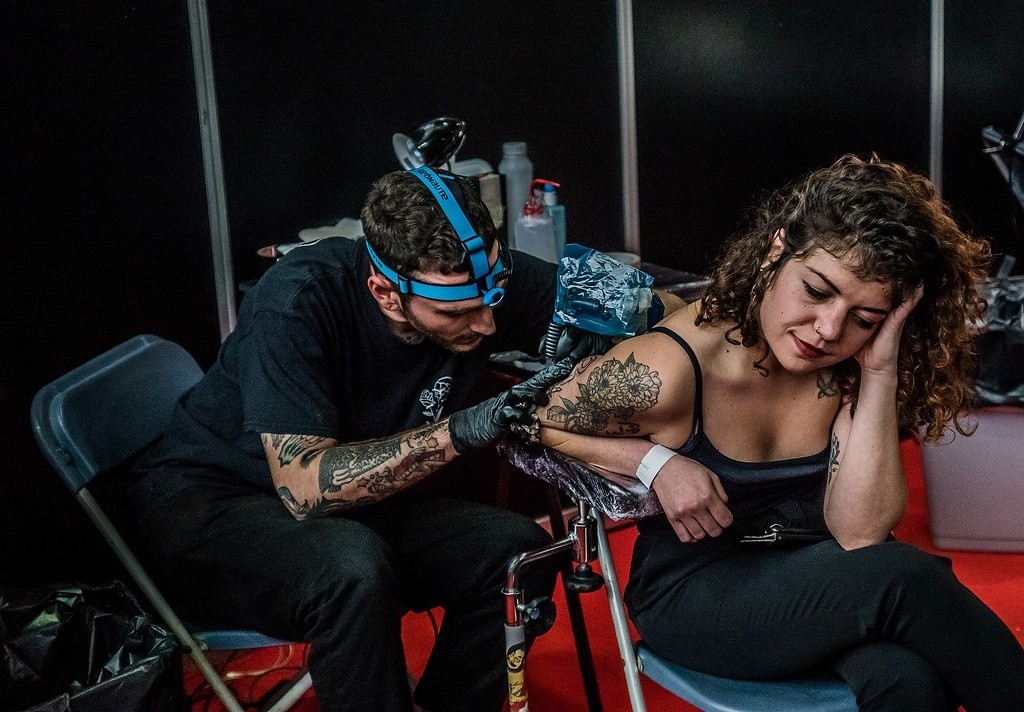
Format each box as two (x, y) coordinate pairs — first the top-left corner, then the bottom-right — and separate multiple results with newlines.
(448, 380), (546, 458)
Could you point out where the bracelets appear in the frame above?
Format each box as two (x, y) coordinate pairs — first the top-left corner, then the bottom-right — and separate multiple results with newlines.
(637, 446), (677, 489)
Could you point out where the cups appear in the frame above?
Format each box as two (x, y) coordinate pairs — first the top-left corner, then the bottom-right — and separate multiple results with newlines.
(606, 253), (641, 271)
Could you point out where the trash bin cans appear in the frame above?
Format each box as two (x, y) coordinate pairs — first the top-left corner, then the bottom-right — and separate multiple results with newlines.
(0, 580), (189, 711)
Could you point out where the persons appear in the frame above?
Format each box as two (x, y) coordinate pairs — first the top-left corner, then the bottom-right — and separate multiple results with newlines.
(138, 168), (687, 712)
(510, 152), (1024, 712)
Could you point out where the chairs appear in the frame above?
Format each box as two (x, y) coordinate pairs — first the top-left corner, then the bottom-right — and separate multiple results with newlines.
(34, 331), (315, 712)
(587, 503), (861, 712)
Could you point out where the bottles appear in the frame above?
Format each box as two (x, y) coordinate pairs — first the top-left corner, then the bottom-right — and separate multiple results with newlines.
(497, 141), (533, 251)
(543, 183), (566, 266)
(514, 177), (560, 266)
